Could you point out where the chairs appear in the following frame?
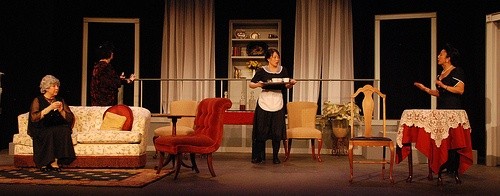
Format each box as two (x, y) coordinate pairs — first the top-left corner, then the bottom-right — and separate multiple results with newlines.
(349, 85), (393, 184)
(156, 96), (233, 180)
(154, 99), (200, 172)
(284, 102), (325, 162)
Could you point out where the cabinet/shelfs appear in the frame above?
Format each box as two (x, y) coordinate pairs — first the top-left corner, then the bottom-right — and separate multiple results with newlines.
(228, 19), (283, 112)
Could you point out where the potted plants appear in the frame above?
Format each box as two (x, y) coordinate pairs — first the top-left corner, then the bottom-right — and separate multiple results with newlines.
(320, 99), (363, 155)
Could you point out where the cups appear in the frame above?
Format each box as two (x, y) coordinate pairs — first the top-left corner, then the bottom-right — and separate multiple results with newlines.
(267, 78), (289, 83)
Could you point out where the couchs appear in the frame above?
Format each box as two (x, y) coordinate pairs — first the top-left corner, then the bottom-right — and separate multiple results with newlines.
(14, 105), (151, 169)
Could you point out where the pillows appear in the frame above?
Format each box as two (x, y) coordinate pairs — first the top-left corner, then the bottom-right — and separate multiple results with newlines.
(102, 104), (134, 132)
(100, 111), (126, 133)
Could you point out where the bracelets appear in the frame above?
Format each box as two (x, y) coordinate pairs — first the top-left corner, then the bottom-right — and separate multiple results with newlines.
(445, 86), (448, 91)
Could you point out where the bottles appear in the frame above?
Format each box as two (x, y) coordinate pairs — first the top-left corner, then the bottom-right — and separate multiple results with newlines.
(231, 45), (248, 55)
(234, 67), (238, 79)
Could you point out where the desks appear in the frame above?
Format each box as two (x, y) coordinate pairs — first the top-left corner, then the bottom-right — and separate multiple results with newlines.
(152, 113), (199, 173)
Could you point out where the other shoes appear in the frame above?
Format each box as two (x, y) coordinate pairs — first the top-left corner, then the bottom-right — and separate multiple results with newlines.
(441, 160), (455, 175)
(272, 156), (281, 164)
(257, 155), (265, 164)
(49, 161), (61, 172)
(41, 164), (50, 174)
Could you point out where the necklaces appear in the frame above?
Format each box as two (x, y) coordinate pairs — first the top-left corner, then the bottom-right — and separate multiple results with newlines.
(442, 66), (454, 76)
(45, 95), (57, 104)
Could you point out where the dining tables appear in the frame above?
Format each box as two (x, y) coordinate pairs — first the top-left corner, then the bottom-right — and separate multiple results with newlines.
(395, 109), (475, 189)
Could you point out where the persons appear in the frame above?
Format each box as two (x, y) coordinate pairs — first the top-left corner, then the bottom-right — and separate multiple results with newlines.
(90, 45), (137, 107)
(415, 47), (472, 176)
(28, 75), (77, 174)
(250, 49), (297, 165)
